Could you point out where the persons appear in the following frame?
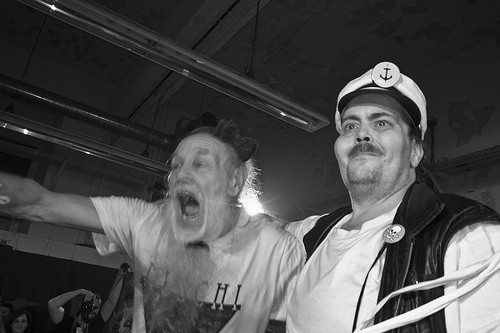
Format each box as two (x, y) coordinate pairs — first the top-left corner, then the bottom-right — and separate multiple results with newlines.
(1, 132), (305, 332)
(253, 61), (500, 333)
(0, 262), (133, 333)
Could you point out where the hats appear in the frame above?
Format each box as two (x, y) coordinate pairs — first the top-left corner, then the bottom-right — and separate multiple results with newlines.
(335, 62), (427, 141)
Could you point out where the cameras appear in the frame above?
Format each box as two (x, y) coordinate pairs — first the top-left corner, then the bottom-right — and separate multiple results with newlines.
(81, 295), (93, 313)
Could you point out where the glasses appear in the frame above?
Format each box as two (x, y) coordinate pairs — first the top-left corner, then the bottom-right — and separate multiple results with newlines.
(13, 319), (27, 325)
